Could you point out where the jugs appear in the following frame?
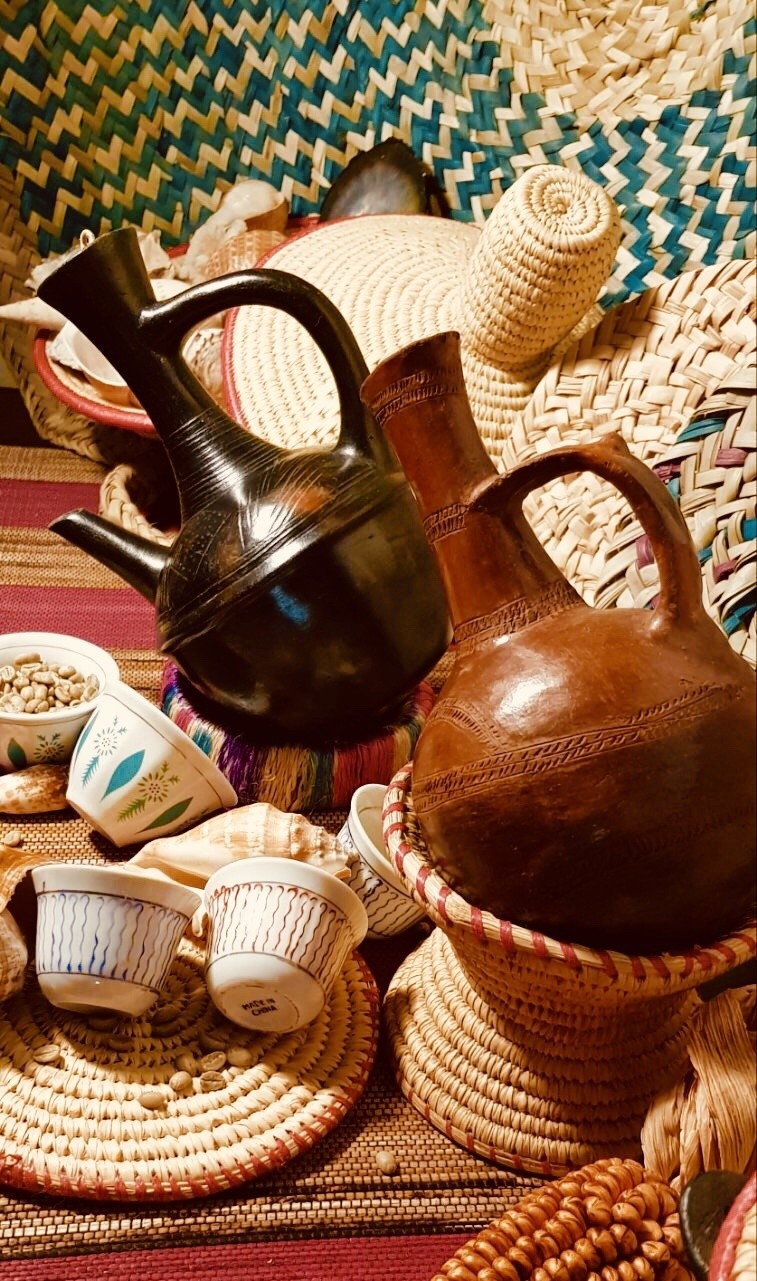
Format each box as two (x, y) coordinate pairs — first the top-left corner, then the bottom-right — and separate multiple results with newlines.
(49, 227), (455, 748)
(359, 329), (757, 952)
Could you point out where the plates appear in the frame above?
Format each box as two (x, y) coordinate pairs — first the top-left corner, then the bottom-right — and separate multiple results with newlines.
(30, 217), (318, 438)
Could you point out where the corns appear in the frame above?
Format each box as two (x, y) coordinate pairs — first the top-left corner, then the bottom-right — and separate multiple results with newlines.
(427, 1157), (698, 1281)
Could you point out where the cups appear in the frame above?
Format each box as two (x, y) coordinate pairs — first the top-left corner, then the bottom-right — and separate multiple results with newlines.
(335, 786), (426, 942)
(64, 682), (237, 847)
(62, 277), (197, 406)
(32, 863), (202, 1015)
(0, 630), (119, 774)
(201, 855), (368, 1035)
